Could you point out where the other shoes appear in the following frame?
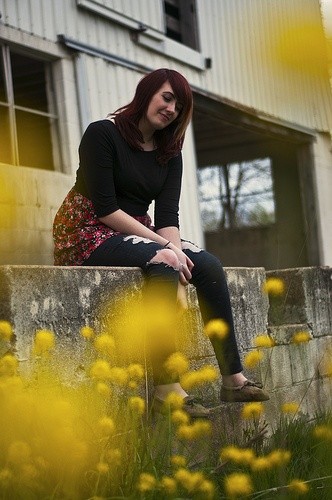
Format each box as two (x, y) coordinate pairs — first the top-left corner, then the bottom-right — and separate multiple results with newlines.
(148, 391), (210, 420)
(217, 378), (270, 403)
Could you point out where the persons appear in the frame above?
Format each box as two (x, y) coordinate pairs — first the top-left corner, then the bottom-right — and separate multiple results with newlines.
(51, 68), (270, 419)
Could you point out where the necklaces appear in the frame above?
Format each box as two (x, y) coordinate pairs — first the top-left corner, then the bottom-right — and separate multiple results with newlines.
(137, 141), (157, 177)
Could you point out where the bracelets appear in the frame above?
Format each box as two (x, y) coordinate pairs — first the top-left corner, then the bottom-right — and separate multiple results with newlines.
(164, 240), (172, 247)
